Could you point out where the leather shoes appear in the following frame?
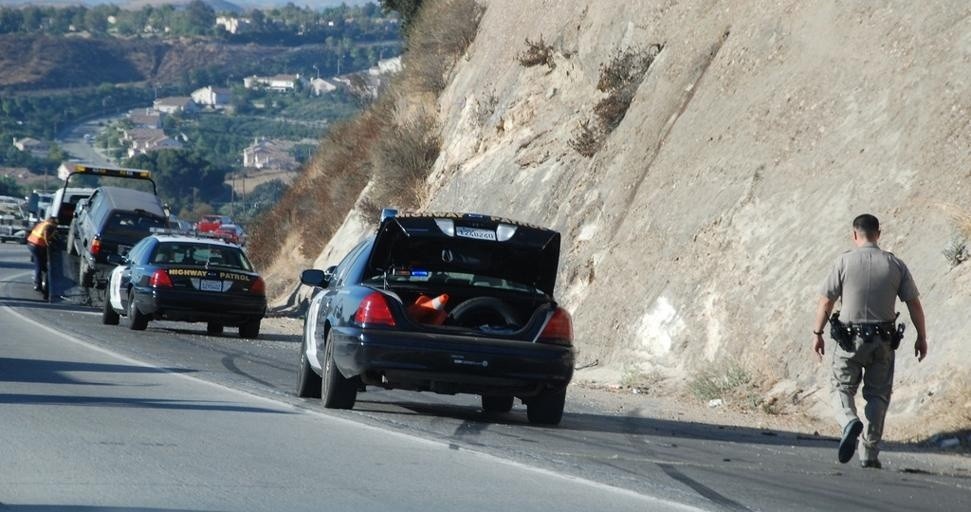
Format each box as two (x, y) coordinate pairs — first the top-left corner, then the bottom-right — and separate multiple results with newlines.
(838, 418), (864, 464)
(861, 458), (882, 469)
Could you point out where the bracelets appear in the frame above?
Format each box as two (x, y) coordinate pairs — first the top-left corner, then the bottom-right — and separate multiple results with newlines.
(813, 330), (823, 335)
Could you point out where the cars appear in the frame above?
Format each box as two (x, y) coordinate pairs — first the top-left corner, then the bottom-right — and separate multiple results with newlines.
(294, 208), (577, 429)
(1, 163), (233, 290)
(214, 224), (248, 246)
(101, 227), (266, 337)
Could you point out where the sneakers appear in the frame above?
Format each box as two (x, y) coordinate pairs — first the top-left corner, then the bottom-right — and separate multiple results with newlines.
(33, 285), (45, 295)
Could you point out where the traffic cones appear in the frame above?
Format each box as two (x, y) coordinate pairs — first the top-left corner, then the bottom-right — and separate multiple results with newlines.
(407, 292), (448, 324)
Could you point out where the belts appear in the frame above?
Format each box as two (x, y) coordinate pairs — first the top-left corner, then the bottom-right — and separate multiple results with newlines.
(842, 320), (898, 336)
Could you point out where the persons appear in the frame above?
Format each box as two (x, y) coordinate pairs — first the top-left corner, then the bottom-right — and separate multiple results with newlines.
(813, 213), (927, 469)
(27, 217), (60, 294)
